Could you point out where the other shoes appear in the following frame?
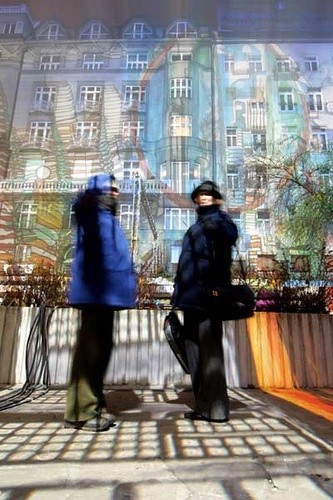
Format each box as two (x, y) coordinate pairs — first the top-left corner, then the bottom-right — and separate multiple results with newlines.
(184, 409), (210, 420)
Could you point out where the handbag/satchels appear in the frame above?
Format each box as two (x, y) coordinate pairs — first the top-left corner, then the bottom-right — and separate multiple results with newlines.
(163, 311), (190, 374)
(210, 284), (255, 320)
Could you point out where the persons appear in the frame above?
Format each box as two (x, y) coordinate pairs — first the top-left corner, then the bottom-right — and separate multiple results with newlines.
(166, 180), (238, 424)
(63, 172), (139, 432)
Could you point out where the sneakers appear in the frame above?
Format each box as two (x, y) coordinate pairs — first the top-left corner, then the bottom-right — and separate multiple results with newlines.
(99, 411), (117, 424)
(63, 415), (109, 431)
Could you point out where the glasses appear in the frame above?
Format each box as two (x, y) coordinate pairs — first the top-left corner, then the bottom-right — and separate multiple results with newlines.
(102, 186), (118, 192)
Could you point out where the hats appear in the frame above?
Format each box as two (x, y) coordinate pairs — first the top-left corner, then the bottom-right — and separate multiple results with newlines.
(191, 181), (223, 203)
